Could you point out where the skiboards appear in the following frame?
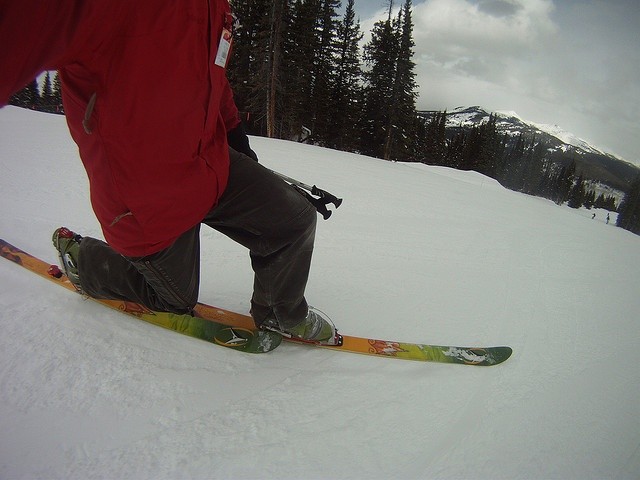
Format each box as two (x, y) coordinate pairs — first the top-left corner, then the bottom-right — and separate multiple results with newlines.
(0, 239), (512, 366)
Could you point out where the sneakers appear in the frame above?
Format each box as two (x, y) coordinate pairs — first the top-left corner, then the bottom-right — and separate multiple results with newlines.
(52, 227), (84, 288)
(250, 309), (332, 341)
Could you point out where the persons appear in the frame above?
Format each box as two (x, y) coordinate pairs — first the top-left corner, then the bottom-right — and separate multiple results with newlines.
(0, 1), (333, 341)
